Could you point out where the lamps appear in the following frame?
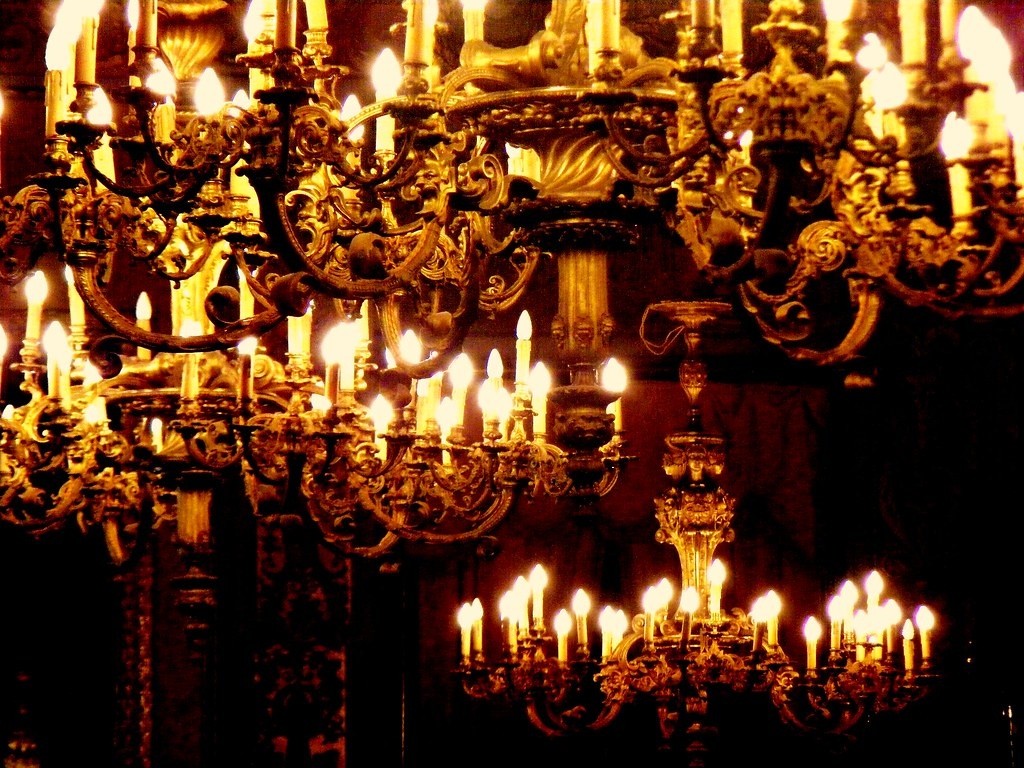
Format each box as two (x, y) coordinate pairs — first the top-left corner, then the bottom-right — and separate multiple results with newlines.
(456, 297), (935, 754)
(1, 0), (1024, 548)
